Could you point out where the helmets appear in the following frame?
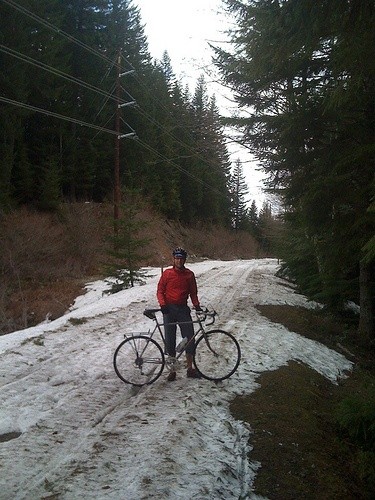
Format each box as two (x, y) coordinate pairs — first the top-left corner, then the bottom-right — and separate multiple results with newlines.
(172, 247), (188, 259)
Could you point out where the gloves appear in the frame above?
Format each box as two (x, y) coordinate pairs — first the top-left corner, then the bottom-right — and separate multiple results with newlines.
(195, 307), (203, 315)
(161, 305), (169, 314)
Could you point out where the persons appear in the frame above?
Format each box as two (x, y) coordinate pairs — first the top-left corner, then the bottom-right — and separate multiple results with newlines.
(156, 247), (202, 381)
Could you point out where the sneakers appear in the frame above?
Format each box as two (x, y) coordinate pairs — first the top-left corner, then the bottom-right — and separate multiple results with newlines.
(187, 368), (201, 378)
(168, 372), (176, 381)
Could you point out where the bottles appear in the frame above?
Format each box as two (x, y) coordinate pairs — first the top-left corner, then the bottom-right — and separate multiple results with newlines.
(175, 336), (188, 353)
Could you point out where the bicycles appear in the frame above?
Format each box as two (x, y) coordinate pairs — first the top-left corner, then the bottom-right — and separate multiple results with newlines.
(113, 307), (241, 386)
